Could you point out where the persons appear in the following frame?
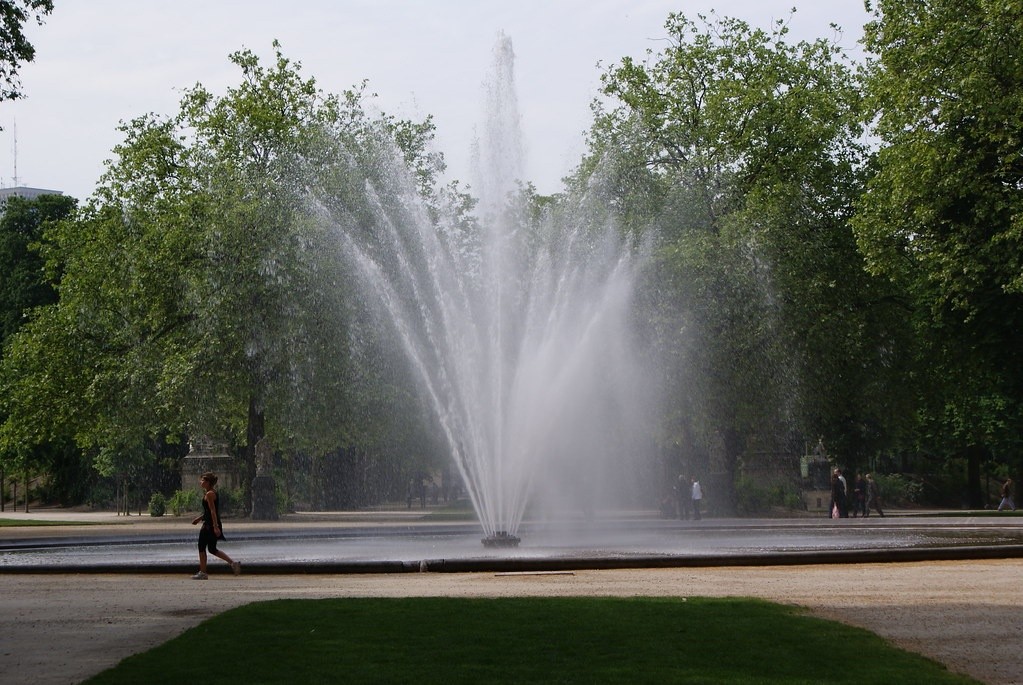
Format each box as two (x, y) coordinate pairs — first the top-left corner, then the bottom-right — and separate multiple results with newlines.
(404, 482), (460, 508)
(672, 475), (702, 520)
(828, 468), (849, 518)
(191, 472), (241, 580)
(998, 479), (1017, 511)
(850, 474), (885, 518)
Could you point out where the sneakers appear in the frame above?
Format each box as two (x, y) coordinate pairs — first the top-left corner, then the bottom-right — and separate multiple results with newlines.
(190, 571), (208, 580)
(232, 561), (242, 575)
